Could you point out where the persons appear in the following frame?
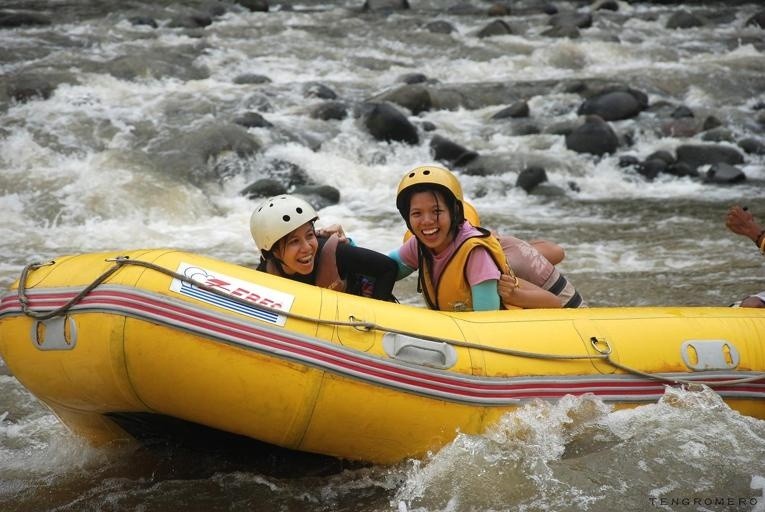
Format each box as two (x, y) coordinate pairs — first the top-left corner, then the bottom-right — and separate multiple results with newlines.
(403, 192), (589, 309)
(312, 163), (527, 314)
(250, 192), (398, 303)
(725, 205), (764, 309)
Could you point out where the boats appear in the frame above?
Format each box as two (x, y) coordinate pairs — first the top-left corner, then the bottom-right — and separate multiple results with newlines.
(0, 249), (764, 469)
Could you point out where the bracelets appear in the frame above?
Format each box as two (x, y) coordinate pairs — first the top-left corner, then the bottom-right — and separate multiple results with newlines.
(759, 239), (764, 254)
(755, 230), (764, 247)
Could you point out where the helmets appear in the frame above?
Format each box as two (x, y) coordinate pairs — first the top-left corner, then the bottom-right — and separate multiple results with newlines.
(250, 194), (318, 261)
(396, 165), (482, 244)
